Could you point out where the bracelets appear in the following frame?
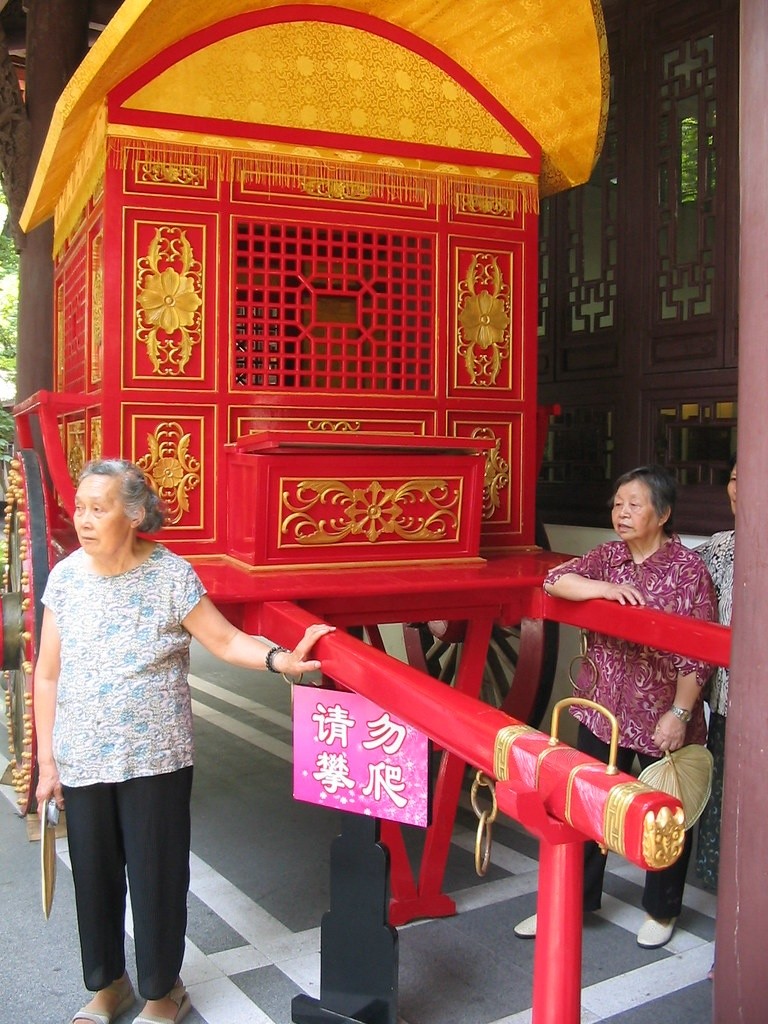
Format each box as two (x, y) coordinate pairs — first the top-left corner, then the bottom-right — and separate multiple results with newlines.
(265, 646), (286, 673)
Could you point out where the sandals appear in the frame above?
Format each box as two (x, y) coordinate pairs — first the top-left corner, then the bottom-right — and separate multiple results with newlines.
(130, 976), (190, 1024)
(72, 970), (135, 1024)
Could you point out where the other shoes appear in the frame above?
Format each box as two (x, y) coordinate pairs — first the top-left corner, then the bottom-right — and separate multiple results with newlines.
(514, 915), (536, 939)
(637, 917), (676, 949)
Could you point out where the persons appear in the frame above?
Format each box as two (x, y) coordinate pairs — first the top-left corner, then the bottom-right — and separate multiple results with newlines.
(513, 464), (719, 950)
(33, 459), (337, 1024)
(548, 456), (736, 889)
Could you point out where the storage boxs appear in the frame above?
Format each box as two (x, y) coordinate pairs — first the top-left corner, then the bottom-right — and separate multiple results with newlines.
(223, 431), (496, 566)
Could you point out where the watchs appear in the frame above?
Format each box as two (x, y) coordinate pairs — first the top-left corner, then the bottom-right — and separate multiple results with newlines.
(671, 705), (691, 722)
